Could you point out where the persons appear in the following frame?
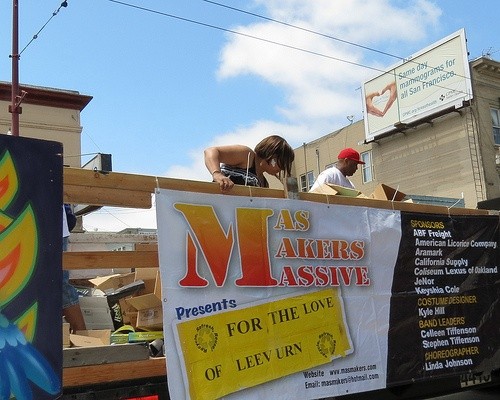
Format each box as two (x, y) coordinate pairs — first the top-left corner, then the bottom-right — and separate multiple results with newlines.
(366, 81), (399, 117)
(203, 135), (295, 193)
(63, 203), (86, 347)
(308, 148), (366, 192)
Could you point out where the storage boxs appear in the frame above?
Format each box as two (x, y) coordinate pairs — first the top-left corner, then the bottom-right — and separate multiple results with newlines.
(62, 242), (163, 348)
(308, 183), (413, 205)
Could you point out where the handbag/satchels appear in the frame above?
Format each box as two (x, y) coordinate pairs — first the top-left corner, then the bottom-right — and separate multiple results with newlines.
(64, 203), (76, 232)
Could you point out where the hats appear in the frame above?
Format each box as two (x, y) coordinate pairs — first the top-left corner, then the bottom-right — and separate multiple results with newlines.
(338, 147), (365, 164)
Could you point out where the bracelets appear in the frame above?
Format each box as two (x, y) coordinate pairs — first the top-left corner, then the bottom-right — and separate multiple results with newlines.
(211, 170), (221, 175)
